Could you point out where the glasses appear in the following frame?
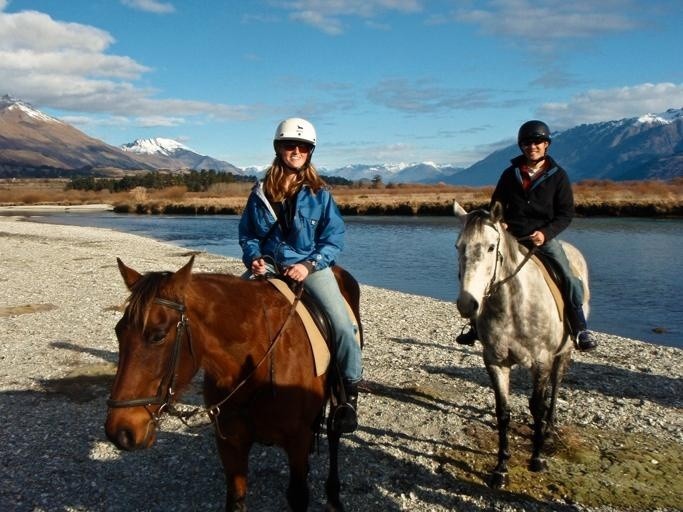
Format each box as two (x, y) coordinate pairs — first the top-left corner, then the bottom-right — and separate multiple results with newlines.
(525, 137), (545, 146)
(283, 140), (311, 153)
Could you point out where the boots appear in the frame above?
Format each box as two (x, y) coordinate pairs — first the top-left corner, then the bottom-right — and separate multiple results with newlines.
(456, 328), (479, 343)
(334, 385), (359, 433)
(572, 305), (597, 350)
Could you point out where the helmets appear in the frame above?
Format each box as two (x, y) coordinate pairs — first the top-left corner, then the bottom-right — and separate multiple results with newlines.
(518, 120), (553, 144)
(275, 117), (317, 145)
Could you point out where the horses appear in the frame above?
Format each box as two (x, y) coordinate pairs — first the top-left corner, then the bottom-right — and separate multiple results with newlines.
(103, 254), (363, 512)
(448, 196), (592, 489)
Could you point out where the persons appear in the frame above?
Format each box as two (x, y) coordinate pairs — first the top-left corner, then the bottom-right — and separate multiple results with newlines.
(239, 117), (363, 433)
(457, 121), (598, 352)
